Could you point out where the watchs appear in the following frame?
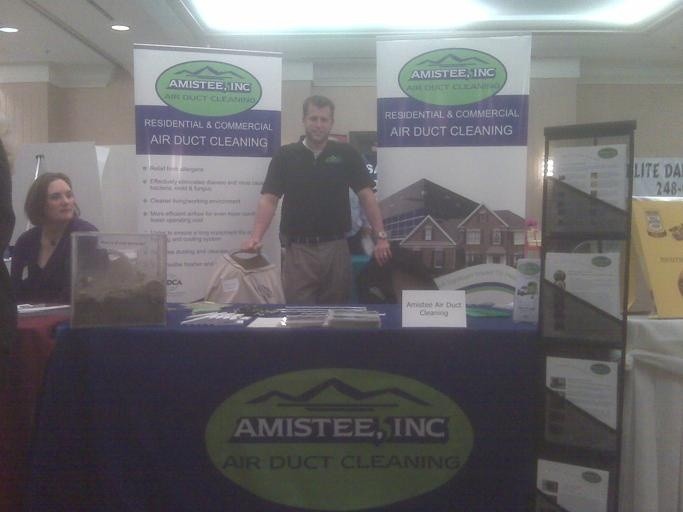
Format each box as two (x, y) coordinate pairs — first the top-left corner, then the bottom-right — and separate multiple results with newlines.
(370, 230), (390, 241)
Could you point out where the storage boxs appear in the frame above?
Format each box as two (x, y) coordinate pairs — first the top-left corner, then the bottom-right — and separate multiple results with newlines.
(70, 231), (168, 328)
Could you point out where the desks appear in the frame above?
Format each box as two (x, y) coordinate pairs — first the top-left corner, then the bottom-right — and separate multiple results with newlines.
(0, 305), (72, 512)
(21, 304), (538, 512)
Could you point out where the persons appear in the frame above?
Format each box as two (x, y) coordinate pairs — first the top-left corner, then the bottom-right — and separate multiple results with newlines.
(0, 140), (17, 353)
(10, 170), (112, 305)
(243, 95), (395, 307)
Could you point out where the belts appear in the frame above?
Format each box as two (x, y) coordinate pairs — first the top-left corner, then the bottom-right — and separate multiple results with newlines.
(288, 234), (345, 243)
